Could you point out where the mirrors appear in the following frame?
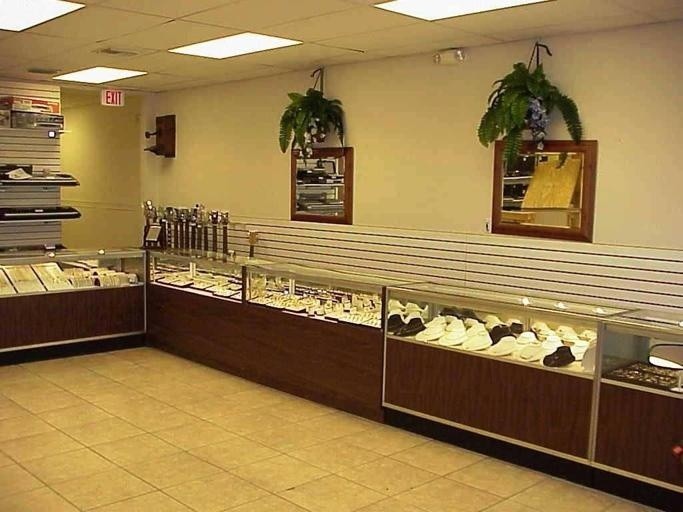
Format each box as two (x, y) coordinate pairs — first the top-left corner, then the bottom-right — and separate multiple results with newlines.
(491, 140), (597, 244)
(290, 147), (354, 225)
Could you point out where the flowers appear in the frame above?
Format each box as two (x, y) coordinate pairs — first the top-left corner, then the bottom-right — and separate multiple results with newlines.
(477, 62), (585, 173)
(279, 88), (344, 166)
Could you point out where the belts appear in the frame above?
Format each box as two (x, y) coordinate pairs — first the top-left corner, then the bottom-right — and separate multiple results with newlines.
(157, 207), (165, 249)
(166, 207), (173, 249)
(203, 211), (209, 251)
(211, 211), (218, 251)
(181, 209), (188, 249)
(196, 211), (202, 250)
(177, 209), (184, 249)
(190, 209), (197, 249)
(249, 232), (255, 257)
(221, 212), (228, 254)
(172, 208), (178, 249)
(144, 200), (157, 247)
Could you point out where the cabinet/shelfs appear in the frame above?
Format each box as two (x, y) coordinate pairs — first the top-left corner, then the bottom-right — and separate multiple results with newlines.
(144, 115), (175, 157)
(382, 285), (683, 512)
(1, 250), (146, 367)
(148, 249), (383, 427)
(0, 126), (62, 258)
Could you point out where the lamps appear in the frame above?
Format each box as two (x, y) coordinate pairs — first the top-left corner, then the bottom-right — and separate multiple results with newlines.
(433, 48), (467, 66)
